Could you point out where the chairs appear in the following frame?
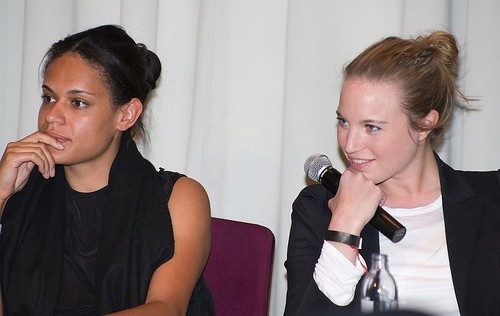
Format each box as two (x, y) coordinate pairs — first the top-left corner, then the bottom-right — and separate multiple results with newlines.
(204, 217), (275, 316)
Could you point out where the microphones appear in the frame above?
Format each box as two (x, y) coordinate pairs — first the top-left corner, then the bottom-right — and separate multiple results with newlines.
(304, 152), (406, 243)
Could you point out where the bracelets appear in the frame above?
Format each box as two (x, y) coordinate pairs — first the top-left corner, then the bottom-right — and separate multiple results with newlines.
(326, 230), (362, 249)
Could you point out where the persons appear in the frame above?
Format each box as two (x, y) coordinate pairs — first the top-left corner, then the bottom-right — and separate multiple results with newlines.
(284, 29), (500, 316)
(1, 23), (215, 316)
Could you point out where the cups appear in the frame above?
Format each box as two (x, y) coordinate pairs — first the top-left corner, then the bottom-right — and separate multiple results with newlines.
(359, 252), (398, 316)
